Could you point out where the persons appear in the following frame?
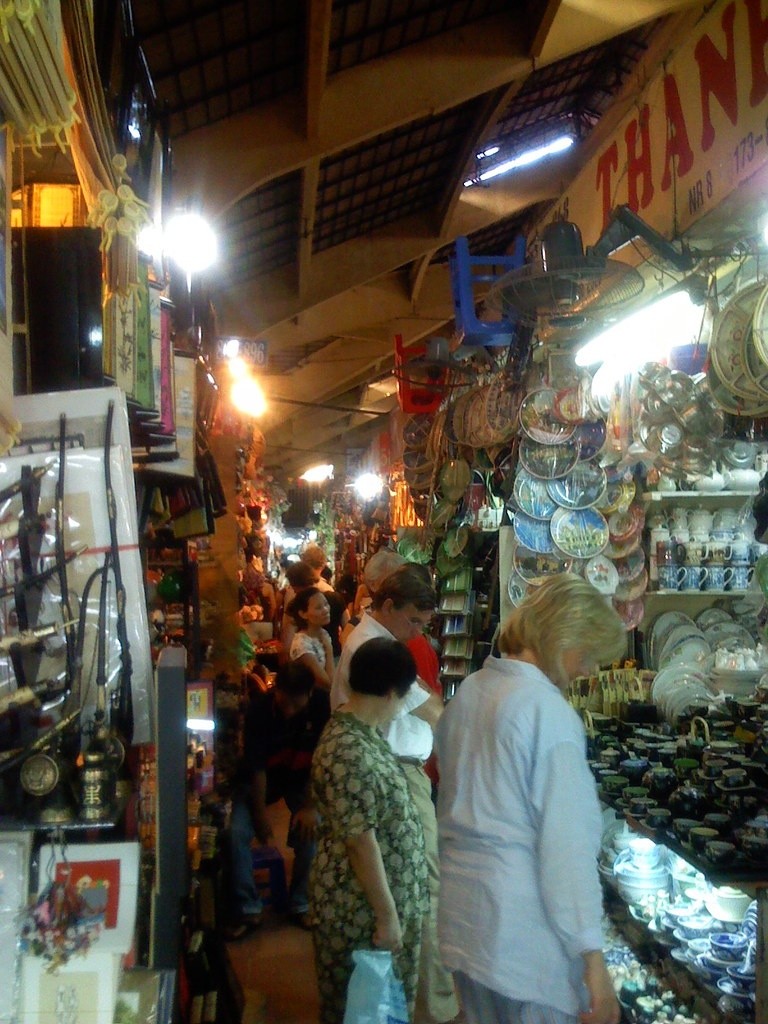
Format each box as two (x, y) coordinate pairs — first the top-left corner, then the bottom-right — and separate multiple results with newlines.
(226, 528), (463, 1024)
(429, 571), (629, 1024)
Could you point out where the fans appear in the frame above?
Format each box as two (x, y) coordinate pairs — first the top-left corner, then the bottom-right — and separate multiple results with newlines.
(485, 211), (647, 328)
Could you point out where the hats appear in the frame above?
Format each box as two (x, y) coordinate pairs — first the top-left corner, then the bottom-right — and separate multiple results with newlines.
(364, 550), (409, 592)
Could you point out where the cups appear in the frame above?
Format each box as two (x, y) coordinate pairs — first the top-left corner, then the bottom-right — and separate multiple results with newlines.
(648, 505), (767, 592)
(576, 734), (767, 1024)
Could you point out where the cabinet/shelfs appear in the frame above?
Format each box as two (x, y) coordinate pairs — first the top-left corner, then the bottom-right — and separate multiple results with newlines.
(625, 424), (768, 663)
(139, 533), (209, 684)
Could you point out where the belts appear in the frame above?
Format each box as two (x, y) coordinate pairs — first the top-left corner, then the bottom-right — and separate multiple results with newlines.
(401, 756), (427, 768)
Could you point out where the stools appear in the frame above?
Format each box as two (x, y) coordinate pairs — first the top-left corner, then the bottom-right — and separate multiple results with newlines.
(446, 231), (531, 345)
(388, 334), (451, 415)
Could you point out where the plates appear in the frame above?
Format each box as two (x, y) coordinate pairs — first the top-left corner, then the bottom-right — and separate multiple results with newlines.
(397, 388), (648, 633)
(649, 610), (766, 728)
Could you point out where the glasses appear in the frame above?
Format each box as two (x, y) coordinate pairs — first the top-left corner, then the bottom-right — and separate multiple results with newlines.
(398, 609), (432, 634)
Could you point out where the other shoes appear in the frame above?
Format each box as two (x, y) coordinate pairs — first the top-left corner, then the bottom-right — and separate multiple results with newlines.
(223, 913), (262, 939)
(292, 911), (320, 929)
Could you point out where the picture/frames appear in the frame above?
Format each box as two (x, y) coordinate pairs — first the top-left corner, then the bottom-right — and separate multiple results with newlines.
(35, 837), (140, 955)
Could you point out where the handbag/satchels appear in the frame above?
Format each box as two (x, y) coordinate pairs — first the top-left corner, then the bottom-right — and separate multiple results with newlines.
(342, 949), (410, 1023)
